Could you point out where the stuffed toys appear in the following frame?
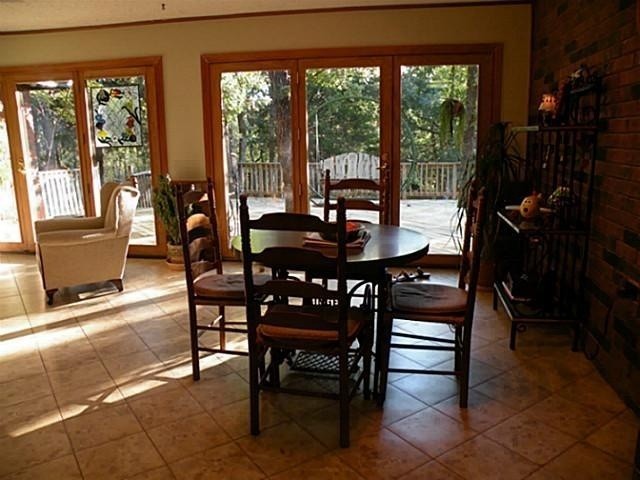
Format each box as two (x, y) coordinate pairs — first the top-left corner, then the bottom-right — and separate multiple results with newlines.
(503, 191), (557, 223)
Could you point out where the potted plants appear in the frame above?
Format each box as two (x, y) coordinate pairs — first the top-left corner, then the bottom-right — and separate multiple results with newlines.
(457, 122), (526, 287)
(151, 174), (202, 262)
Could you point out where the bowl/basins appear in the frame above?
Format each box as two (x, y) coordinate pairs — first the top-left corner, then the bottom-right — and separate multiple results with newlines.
(317, 222), (366, 244)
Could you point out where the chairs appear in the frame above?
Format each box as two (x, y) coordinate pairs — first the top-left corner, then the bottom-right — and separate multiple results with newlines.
(304, 169), (392, 306)
(239, 193), (373, 448)
(35, 181), (141, 306)
(176, 175), (296, 390)
(375, 184), (488, 410)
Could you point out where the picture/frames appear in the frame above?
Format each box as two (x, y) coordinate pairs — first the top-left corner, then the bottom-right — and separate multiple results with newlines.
(90, 84), (143, 149)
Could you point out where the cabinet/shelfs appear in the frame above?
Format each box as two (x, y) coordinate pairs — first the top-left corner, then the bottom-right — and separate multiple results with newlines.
(493, 84), (602, 351)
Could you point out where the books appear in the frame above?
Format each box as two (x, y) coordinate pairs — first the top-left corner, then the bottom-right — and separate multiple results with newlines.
(304, 226), (373, 248)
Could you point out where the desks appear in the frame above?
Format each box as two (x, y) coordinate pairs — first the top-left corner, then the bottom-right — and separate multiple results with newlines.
(231, 224), (429, 400)
